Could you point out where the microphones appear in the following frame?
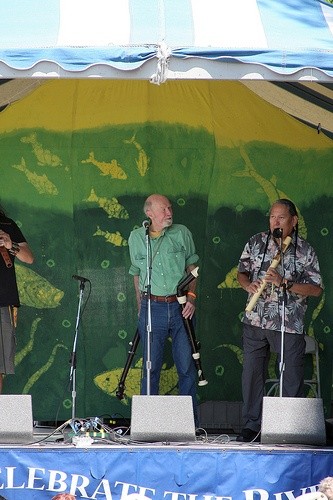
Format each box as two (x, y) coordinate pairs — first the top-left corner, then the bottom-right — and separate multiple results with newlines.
(273, 227), (283, 238)
(143, 220), (150, 228)
(72, 275), (89, 282)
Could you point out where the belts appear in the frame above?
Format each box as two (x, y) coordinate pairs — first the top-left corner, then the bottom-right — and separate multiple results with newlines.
(142, 292), (177, 303)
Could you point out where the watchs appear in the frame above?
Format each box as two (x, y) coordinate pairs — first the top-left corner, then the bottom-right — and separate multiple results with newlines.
(286, 281), (294, 290)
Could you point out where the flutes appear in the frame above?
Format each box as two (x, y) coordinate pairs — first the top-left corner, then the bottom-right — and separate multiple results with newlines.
(176, 266), (208, 386)
(0, 246), (13, 268)
(116, 328), (141, 400)
(245, 235), (293, 312)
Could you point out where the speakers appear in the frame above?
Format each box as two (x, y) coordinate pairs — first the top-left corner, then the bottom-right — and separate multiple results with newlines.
(131, 394), (196, 442)
(0, 394), (33, 444)
(260, 396), (326, 445)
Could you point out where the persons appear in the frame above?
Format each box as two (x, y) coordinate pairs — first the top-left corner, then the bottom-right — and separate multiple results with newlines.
(236, 198), (325, 442)
(0, 204), (34, 394)
(128, 193), (200, 435)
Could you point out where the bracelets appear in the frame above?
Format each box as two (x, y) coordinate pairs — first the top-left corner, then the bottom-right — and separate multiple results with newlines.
(9, 242), (20, 256)
(187, 291), (197, 299)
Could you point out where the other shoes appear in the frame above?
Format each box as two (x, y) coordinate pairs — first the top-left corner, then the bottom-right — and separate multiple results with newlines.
(237, 429), (261, 442)
